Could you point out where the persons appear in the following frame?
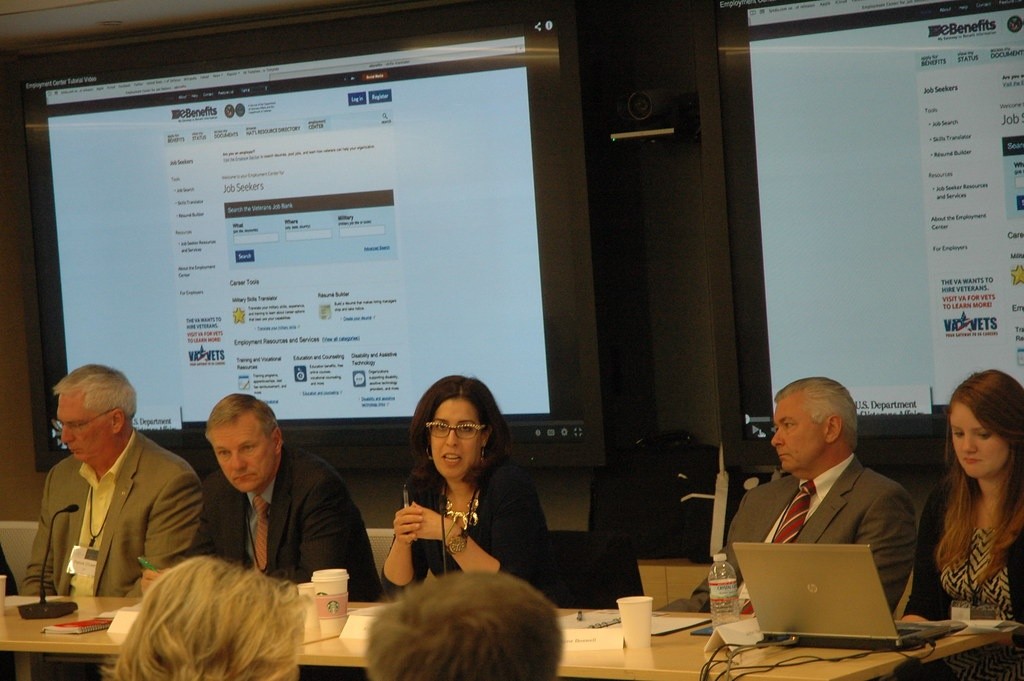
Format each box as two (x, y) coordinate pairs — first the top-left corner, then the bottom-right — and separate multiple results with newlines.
(102, 556), (299, 681)
(141, 394), (387, 681)
(0, 364), (203, 598)
(380, 376), (552, 609)
(367, 567), (565, 681)
(902, 370), (1024, 681)
(655, 376), (916, 616)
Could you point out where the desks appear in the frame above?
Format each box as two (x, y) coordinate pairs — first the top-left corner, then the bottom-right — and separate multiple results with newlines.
(0, 594), (1012, 681)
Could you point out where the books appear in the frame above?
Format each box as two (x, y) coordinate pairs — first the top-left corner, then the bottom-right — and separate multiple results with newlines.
(44, 618), (112, 635)
(560, 610), (713, 638)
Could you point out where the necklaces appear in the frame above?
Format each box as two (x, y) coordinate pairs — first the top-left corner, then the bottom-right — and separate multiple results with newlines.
(445, 494), (478, 531)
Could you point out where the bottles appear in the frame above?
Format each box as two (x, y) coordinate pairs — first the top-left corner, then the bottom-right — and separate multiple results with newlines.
(709, 554), (739, 632)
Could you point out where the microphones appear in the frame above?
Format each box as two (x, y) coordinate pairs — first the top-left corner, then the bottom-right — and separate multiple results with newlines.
(437, 495), (448, 574)
(17, 504), (79, 619)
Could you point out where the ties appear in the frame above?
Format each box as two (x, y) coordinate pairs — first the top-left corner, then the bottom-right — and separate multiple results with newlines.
(252, 495), (271, 574)
(741, 479), (815, 616)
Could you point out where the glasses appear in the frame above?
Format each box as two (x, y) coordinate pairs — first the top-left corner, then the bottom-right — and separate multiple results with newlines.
(51, 407), (118, 432)
(425, 422), (485, 438)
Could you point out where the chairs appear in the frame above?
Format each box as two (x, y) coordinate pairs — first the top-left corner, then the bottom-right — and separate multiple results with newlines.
(0, 521), (39, 594)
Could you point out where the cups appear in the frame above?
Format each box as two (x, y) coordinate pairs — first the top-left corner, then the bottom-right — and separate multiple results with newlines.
(0, 575), (7, 617)
(617, 595), (654, 651)
(297, 568), (349, 643)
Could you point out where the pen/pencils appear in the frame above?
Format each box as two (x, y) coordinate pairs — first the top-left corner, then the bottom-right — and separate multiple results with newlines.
(403, 484), (409, 508)
(577, 610), (582, 621)
(136, 557), (157, 572)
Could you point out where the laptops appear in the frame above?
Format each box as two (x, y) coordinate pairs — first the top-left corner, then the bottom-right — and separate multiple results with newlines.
(733, 542), (968, 652)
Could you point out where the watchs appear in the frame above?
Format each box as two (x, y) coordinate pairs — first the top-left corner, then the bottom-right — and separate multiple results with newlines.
(447, 529), (471, 555)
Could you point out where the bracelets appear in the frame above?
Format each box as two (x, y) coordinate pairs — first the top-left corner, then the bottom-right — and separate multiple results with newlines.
(445, 522), (456, 539)
